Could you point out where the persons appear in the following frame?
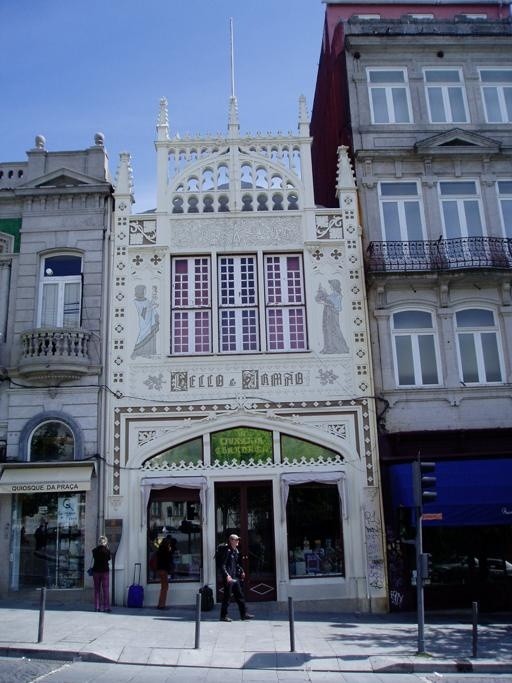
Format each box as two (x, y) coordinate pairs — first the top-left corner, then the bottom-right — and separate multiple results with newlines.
(219, 533), (255, 621)
(315, 277), (350, 353)
(158, 539), (172, 607)
(131, 282), (160, 357)
(92, 535), (113, 612)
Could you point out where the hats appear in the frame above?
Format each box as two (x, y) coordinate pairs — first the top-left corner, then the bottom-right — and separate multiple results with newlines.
(229, 534), (241, 541)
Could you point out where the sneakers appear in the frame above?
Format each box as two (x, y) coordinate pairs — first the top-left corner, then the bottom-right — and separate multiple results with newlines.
(95, 608), (112, 612)
(220, 617), (232, 622)
(241, 613), (254, 621)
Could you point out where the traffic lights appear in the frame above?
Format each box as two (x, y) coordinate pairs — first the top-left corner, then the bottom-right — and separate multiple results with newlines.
(411, 459), (437, 509)
(187, 500), (197, 520)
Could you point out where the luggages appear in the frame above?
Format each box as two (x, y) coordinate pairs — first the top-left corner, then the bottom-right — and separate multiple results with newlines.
(127, 563), (144, 608)
(199, 568), (214, 613)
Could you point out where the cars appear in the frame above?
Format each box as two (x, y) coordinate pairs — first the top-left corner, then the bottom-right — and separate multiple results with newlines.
(432, 553), (511, 585)
(46, 525), (80, 543)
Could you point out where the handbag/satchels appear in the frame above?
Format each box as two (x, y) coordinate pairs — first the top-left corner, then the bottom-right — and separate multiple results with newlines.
(87, 568), (93, 576)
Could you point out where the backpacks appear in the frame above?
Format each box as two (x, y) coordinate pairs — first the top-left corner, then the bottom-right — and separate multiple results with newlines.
(215, 543), (233, 568)
